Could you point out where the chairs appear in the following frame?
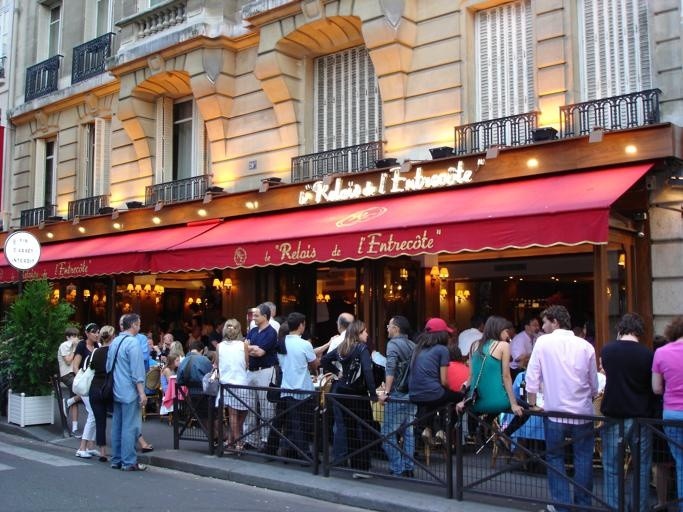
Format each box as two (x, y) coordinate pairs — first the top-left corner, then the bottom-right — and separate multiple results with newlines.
(143, 366), (165, 423)
(320, 374), (333, 407)
(593, 396), (632, 479)
(490, 436), (529, 469)
(424, 440), (447, 465)
(371, 401), (385, 420)
(169, 412), (192, 426)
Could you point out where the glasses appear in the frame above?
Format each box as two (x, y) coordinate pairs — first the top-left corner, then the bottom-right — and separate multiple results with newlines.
(387, 325), (396, 328)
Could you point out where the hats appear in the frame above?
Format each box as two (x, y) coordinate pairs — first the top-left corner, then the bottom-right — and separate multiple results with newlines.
(427, 318), (455, 333)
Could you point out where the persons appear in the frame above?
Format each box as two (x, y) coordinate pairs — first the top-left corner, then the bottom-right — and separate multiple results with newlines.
(177, 341), (228, 449)
(379, 315), (417, 477)
(457, 316), (484, 356)
(650, 335), (675, 512)
(144, 337), (161, 395)
(249, 301), (280, 340)
(147, 302), (223, 360)
(57, 327), (83, 439)
(244, 304), (278, 453)
(73, 322), (101, 459)
(600, 310), (651, 512)
(160, 352), (180, 394)
(652, 315), (683, 511)
(455, 316), (524, 458)
(216, 319), (249, 457)
(509, 318), (540, 385)
(320, 319), (380, 479)
(266, 320), (340, 464)
(106, 313), (147, 471)
(276, 312), (320, 468)
(408, 317), (453, 448)
(525, 304), (599, 511)
(89, 325), (153, 463)
(115, 299), (132, 314)
(326, 312), (355, 354)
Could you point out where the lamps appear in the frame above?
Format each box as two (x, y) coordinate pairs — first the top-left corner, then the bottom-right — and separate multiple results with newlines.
(463, 290), (471, 298)
(188, 297), (193, 305)
(84, 290), (90, 298)
(160, 286), (164, 293)
(143, 284), (151, 294)
(154, 284), (161, 296)
(440, 288), (447, 299)
(127, 284), (134, 293)
(457, 289), (463, 304)
(224, 278), (232, 295)
(196, 298), (202, 304)
(71, 290), (77, 299)
(325, 294), (331, 302)
(134, 285), (142, 294)
(617, 252), (625, 270)
(439, 267), (450, 280)
(430, 265), (440, 280)
(212, 278), (220, 291)
(318, 294), (323, 302)
(399, 268), (409, 281)
(360, 284), (364, 296)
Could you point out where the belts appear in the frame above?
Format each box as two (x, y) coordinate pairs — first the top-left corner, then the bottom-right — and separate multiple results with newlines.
(247, 366), (272, 371)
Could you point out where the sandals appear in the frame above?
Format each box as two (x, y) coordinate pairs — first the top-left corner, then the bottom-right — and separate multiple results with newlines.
(235, 446), (248, 456)
(224, 441), (235, 449)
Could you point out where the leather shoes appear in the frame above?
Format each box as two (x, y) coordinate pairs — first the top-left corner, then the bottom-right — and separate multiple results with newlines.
(99, 456), (109, 462)
(243, 442), (254, 449)
(407, 471), (414, 477)
(258, 442), (270, 451)
(143, 446), (154, 452)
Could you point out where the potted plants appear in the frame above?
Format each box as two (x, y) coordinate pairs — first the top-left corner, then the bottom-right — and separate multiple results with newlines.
(0, 273), (82, 428)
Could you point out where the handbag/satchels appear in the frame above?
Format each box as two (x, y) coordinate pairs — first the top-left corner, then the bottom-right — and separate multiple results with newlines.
(267, 357), (280, 402)
(100, 335), (130, 401)
(464, 340), (496, 406)
(397, 349), (412, 394)
(501, 374), (531, 435)
(345, 344), (367, 394)
(202, 368), (219, 397)
(71, 348), (97, 397)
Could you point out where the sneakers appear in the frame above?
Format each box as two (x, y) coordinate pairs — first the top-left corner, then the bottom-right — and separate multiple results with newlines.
(63, 398), (70, 417)
(112, 463), (121, 469)
(122, 464), (147, 471)
(422, 428), (434, 448)
(496, 436), (513, 451)
(546, 504), (556, 512)
(352, 468), (374, 478)
(474, 438), (486, 455)
(87, 449), (100, 456)
(76, 449), (92, 458)
(70, 430), (83, 438)
(435, 431), (447, 448)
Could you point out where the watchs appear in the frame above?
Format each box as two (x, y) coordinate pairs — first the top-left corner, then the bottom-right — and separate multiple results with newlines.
(529, 404), (536, 407)
(384, 391), (389, 396)
